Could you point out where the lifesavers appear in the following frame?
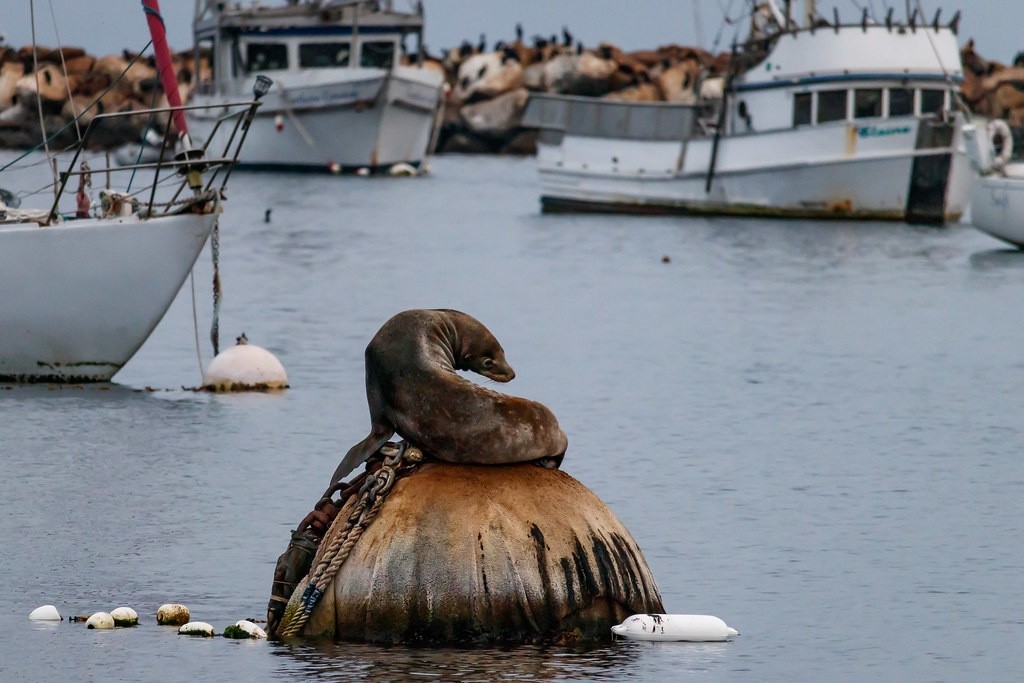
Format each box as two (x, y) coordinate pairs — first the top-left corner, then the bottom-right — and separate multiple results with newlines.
(986, 118), (1013, 169)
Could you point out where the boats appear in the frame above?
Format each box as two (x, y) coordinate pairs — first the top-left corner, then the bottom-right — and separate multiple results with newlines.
(971, 170), (1024, 250)
(514, 0), (991, 222)
(115, 136), (173, 169)
(0, 1), (273, 384)
(174, 1), (450, 172)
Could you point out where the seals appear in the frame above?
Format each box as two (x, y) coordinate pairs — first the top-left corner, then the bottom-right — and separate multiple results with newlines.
(330, 308), (568, 485)
